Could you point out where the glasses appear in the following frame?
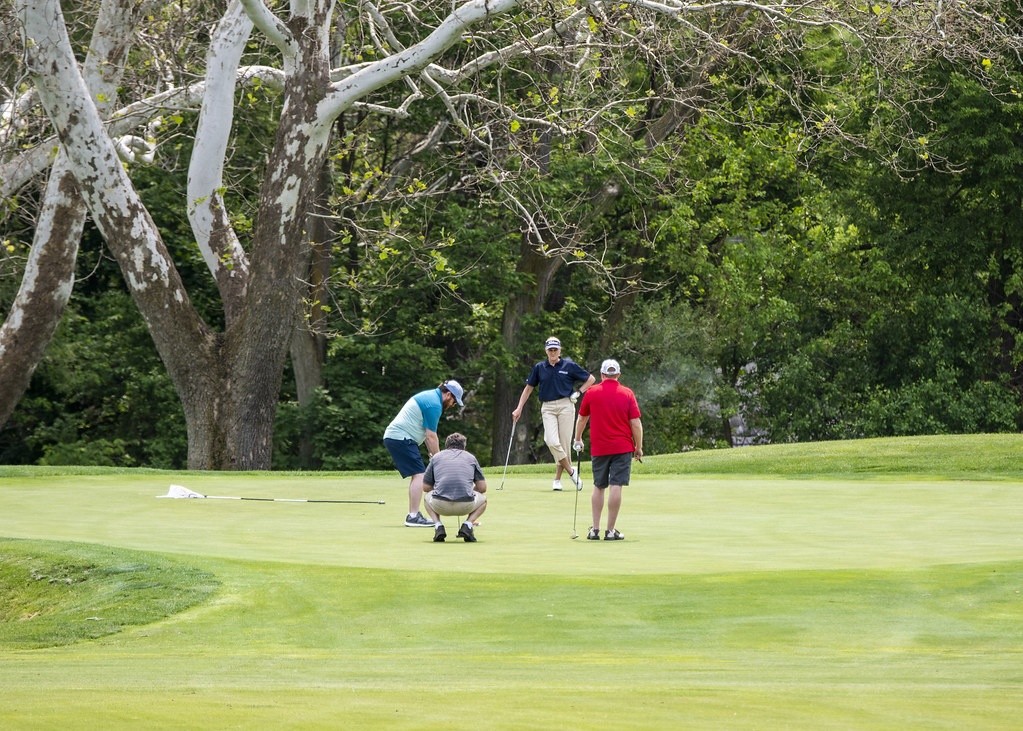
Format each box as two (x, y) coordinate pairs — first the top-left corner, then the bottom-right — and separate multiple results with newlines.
(548, 349), (558, 352)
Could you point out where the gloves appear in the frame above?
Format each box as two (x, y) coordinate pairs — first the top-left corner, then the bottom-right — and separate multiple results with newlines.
(569, 392), (580, 404)
(572, 439), (584, 452)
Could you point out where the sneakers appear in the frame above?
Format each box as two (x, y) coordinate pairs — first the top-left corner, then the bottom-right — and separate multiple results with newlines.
(587, 527), (600, 539)
(553, 480), (562, 490)
(570, 469), (583, 491)
(433, 525), (446, 541)
(458, 524), (477, 542)
(405, 511), (435, 527)
(604, 529), (625, 540)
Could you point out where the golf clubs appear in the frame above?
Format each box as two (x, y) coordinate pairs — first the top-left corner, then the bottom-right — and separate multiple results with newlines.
(570, 445), (580, 541)
(495, 419), (515, 490)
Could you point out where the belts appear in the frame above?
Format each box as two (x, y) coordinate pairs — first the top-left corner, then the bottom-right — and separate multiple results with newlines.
(433, 496), (474, 502)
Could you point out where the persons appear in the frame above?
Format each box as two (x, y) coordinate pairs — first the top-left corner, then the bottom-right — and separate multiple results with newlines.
(383, 380), (463, 527)
(573, 359), (643, 540)
(512, 337), (596, 491)
(422, 433), (487, 542)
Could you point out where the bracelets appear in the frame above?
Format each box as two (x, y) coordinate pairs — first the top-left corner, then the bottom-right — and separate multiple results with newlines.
(576, 390), (582, 396)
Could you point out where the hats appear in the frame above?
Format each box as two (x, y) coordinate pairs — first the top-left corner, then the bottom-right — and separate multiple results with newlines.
(600, 359), (620, 374)
(444, 380), (464, 407)
(545, 336), (561, 350)
